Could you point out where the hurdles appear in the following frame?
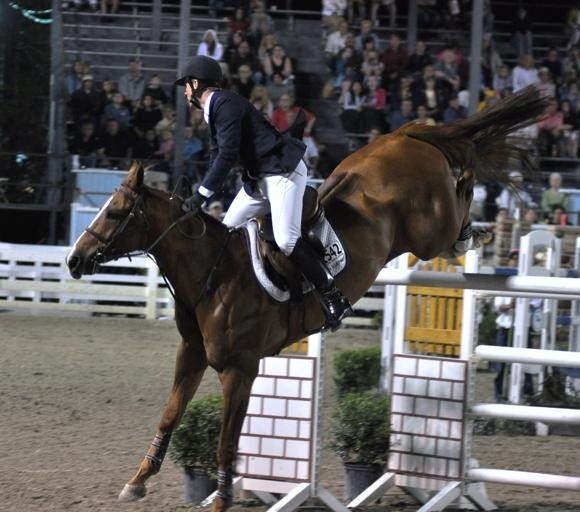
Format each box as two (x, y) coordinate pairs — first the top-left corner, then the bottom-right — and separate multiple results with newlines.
(199, 264), (580, 512)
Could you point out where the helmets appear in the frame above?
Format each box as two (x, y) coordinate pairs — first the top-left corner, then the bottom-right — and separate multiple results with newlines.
(173, 56), (224, 89)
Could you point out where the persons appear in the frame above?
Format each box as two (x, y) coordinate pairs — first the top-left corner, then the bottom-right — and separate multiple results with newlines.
(472, 167), (580, 405)
(65, 57), (236, 210)
(72, 0), (118, 13)
(174, 55), (353, 334)
(197, 3), (318, 174)
(319, 2), (579, 156)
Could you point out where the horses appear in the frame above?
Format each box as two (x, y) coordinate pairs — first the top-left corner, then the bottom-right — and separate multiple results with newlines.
(64, 84), (556, 512)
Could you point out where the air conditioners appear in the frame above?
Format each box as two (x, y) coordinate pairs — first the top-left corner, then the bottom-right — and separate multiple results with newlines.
(168, 393), (224, 504)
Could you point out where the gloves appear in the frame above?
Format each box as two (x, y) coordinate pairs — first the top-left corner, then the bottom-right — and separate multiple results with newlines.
(183, 192), (208, 213)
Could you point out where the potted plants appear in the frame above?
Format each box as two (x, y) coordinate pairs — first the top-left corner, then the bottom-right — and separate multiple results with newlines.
(324, 389), (393, 502)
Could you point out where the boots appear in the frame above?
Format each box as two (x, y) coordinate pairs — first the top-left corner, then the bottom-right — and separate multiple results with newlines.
(288, 237), (353, 333)
